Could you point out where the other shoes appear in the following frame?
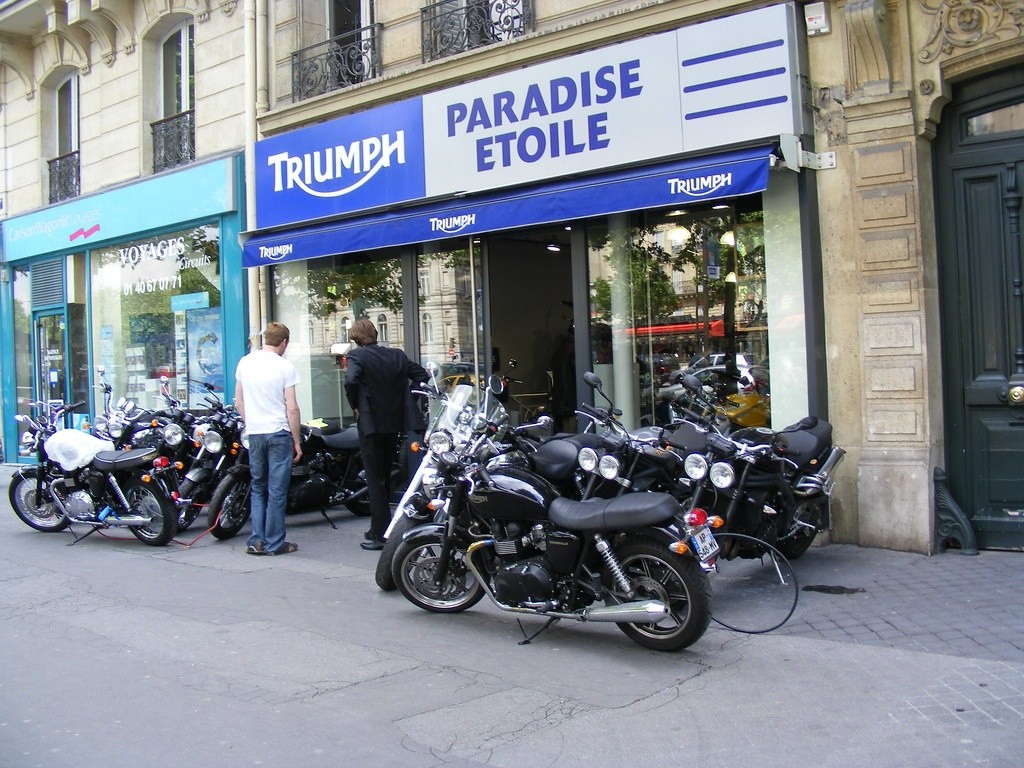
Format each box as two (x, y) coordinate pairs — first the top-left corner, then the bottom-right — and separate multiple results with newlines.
(247, 539), (264, 553)
(265, 542), (298, 556)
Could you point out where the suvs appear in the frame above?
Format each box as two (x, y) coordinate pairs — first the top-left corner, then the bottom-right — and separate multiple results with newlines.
(669, 352), (764, 387)
(637, 353), (681, 385)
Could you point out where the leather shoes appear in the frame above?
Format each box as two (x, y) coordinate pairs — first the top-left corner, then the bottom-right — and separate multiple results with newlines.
(361, 538), (385, 551)
(364, 531), (376, 539)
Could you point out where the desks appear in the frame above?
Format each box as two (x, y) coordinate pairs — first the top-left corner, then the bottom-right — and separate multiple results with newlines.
(510, 393), (549, 422)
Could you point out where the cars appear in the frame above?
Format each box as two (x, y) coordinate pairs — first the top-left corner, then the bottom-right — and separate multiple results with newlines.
(706, 389), (771, 428)
(657, 366), (769, 399)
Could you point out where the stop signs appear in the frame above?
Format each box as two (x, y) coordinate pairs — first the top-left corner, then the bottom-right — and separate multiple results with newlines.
(449, 349), (455, 355)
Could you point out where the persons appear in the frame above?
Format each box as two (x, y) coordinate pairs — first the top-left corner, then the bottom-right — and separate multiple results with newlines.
(344, 321), (429, 539)
(237, 323), (302, 556)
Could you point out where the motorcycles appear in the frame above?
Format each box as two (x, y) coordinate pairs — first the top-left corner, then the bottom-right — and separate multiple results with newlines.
(9, 399), (191, 548)
(91, 361), (848, 592)
(389, 374), (713, 650)
